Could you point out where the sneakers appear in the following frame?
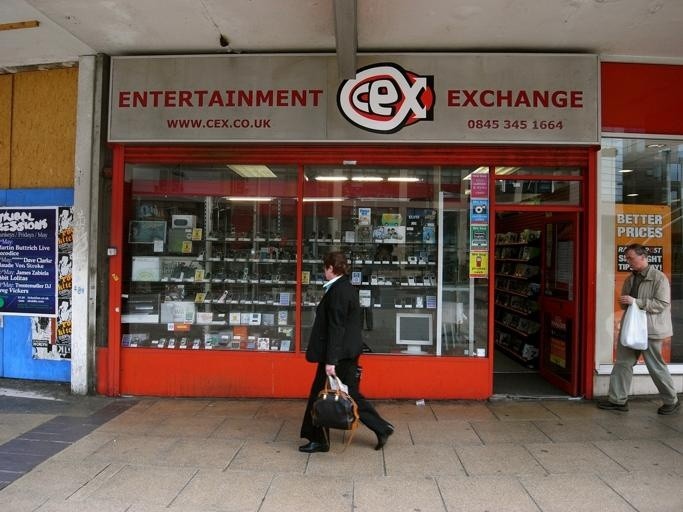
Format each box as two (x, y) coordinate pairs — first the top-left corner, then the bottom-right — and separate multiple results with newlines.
(657, 397), (680, 415)
(598, 400), (628, 412)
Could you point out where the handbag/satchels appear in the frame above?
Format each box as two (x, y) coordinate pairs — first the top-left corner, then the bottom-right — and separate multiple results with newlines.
(311, 375), (360, 454)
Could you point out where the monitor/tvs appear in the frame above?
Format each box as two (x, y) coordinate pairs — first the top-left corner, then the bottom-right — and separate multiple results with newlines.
(396, 312), (433, 355)
(128, 220), (168, 256)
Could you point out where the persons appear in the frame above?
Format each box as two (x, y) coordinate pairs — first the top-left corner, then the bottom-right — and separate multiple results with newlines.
(298, 250), (396, 454)
(596, 243), (681, 415)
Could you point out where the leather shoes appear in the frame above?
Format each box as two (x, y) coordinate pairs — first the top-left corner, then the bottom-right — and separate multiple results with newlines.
(375, 422), (395, 451)
(299, 439), (329, 453)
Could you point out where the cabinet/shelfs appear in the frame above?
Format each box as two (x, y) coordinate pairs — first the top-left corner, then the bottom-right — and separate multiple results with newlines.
(494, 239), (542, 369)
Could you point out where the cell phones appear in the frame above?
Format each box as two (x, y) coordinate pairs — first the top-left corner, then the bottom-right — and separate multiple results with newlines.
(476, 256), (481, 267)
